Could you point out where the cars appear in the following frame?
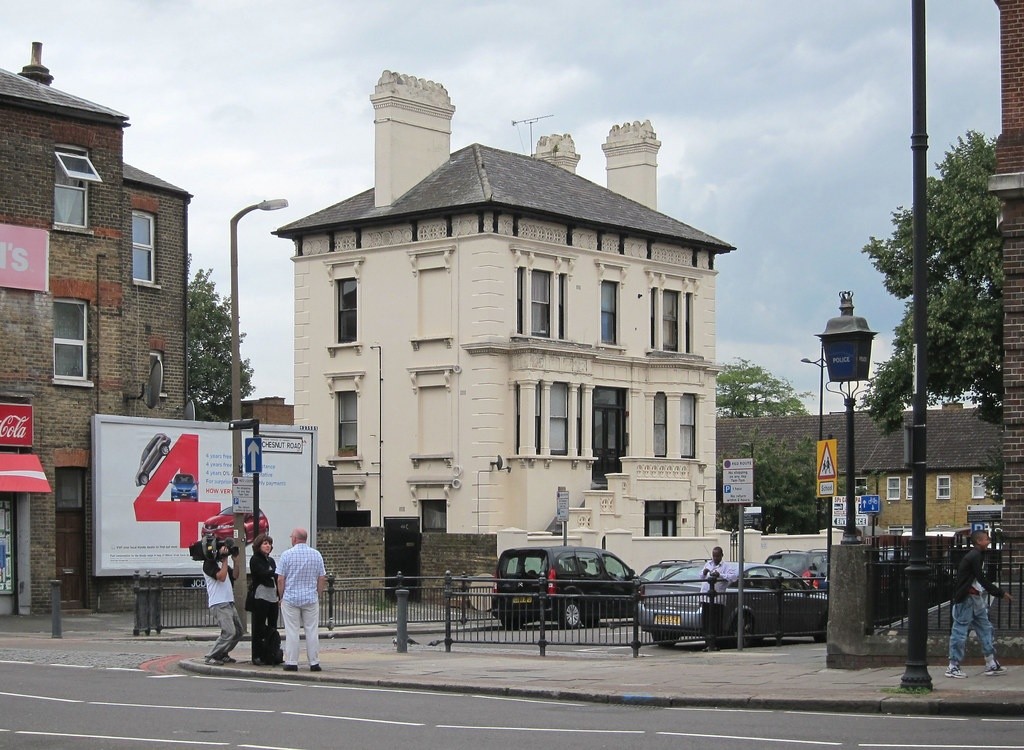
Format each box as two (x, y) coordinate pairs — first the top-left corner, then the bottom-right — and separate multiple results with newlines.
(876, 549), (957, 618)
(201, 504), (270, 544)
(135, 432), (171, 487)
(639, 549), (829, 649)
(169, 473), (200, 502)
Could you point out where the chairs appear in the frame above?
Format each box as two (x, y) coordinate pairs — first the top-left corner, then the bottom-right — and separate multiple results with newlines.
(575, 560), (592, 575)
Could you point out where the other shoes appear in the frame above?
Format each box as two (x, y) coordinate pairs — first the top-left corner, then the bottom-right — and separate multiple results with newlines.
(702, 646), (720, 652)
(310, 664), (321, 671)
(253, 657), (261, 665)
(283, 664), (298, 670)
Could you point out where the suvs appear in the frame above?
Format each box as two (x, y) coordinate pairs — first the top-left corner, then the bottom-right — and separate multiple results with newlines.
(492, 544), (649, 630)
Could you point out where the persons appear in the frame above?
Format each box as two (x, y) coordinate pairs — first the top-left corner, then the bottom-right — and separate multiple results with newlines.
(245, 535), (285, 665)
(945, 530), (1015, 679)
(580, 561), (592, 576)
(275, 528), (326, 672)
(802, 565), (828, 594)
(202, 537), (244, 666)
(700, 547), (737, 652)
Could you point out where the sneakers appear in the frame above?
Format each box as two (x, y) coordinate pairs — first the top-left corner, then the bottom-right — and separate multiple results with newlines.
(945, 663), (968, 678)
(204, 656), (236, 665)
(984, 660), (1007, 675)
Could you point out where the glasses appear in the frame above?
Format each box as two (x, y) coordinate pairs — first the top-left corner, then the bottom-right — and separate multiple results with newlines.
(290, 536), (292, 537)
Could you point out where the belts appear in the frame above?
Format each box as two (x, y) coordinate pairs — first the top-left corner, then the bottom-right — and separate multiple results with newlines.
(969, 587), (979, 595)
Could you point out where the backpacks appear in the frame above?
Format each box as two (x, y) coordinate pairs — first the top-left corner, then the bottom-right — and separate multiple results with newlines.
(264, 625), (283, 664)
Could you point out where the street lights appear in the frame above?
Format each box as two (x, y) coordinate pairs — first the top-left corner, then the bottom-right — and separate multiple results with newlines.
(227, 198), (289, 628)
(813, 290), (881, 544)
(801, 339), (830, 533)
